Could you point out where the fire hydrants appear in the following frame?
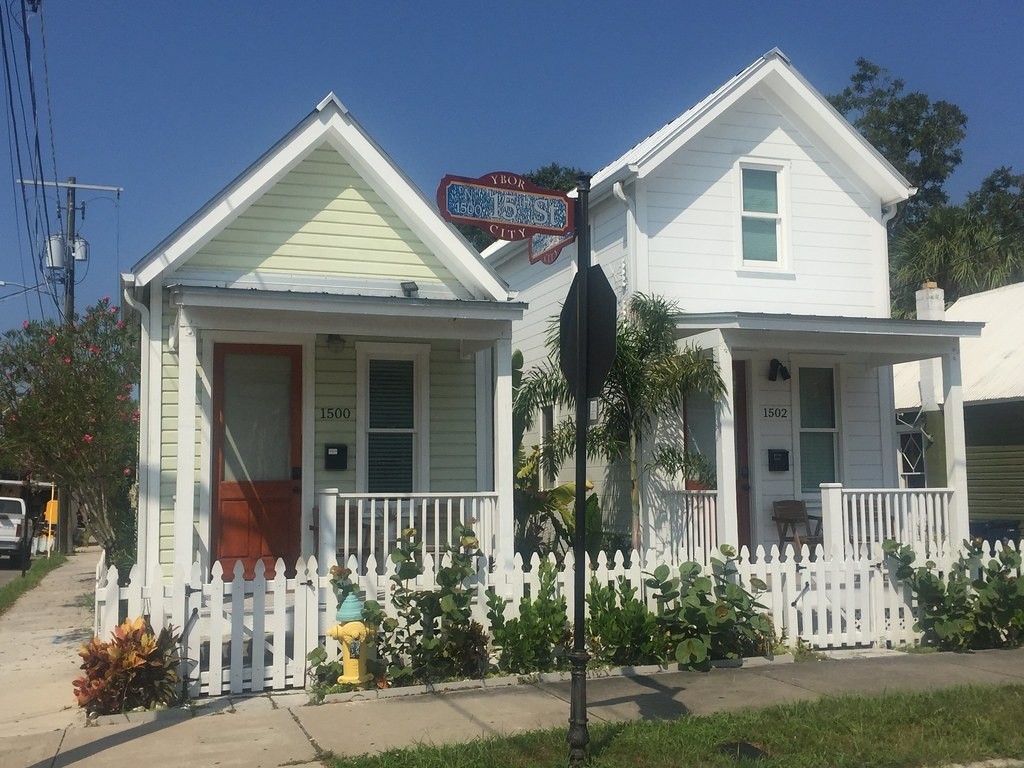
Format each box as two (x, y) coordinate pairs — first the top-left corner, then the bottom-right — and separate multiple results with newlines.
(327, 592), (379, 684)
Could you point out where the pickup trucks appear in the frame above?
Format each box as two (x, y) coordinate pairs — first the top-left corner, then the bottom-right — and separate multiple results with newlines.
(0, 497), (33, 569)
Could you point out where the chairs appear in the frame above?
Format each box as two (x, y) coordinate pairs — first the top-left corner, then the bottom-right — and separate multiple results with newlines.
(772, 500), (822, 562)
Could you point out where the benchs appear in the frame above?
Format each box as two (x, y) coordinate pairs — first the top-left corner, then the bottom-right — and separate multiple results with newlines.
(842, 500), (895, 545)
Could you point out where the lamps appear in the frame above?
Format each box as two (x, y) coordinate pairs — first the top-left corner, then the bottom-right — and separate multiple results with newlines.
(400, 281), (419, 297)
(767, 358), (791, 381)
